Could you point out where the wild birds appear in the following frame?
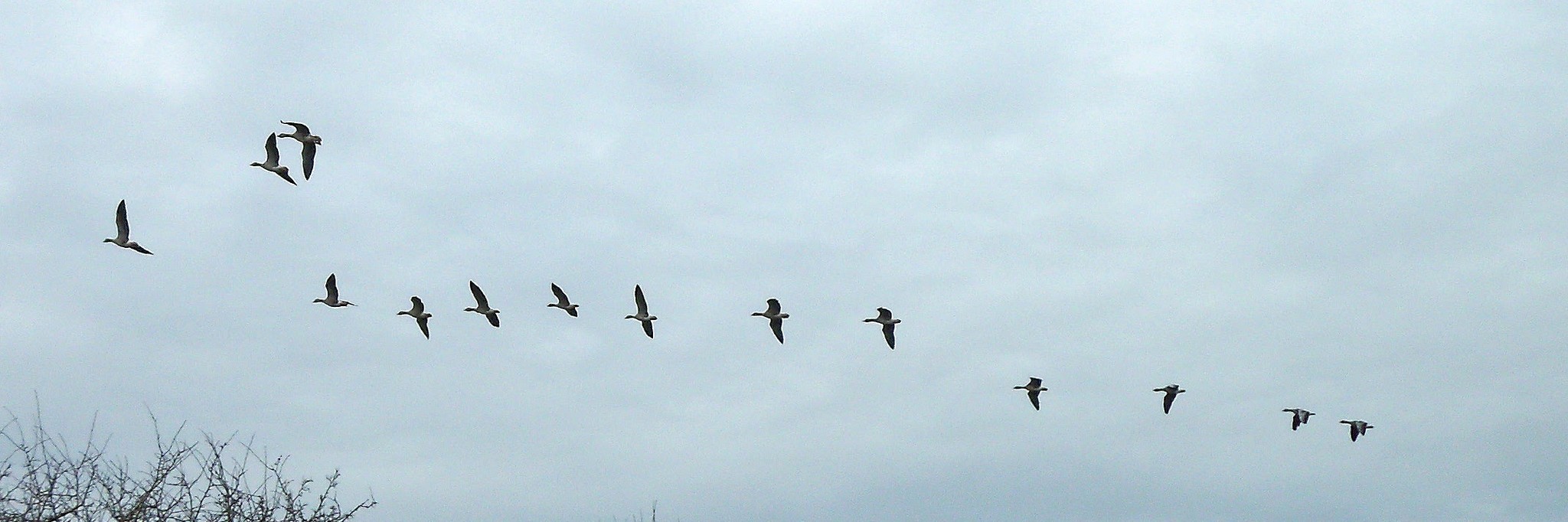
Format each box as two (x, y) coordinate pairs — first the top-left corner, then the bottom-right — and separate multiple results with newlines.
(1013, 377), (1048, 410)
(463, 281), (500, 328)
(102, 199), (153, 255)
(862, 307), (901, 350)
(250, 120), (322, 186)
(751, 299), (790, 344)
(1339, 420), (1374, 442)
(1153, 383), (1186, 414)
(624, 284), (658, 339)
(547, 282), (579, 317)
(397, 296), (433, 340)
(312, 273), (359, 307)
(1282, 407), (1316, 431)
